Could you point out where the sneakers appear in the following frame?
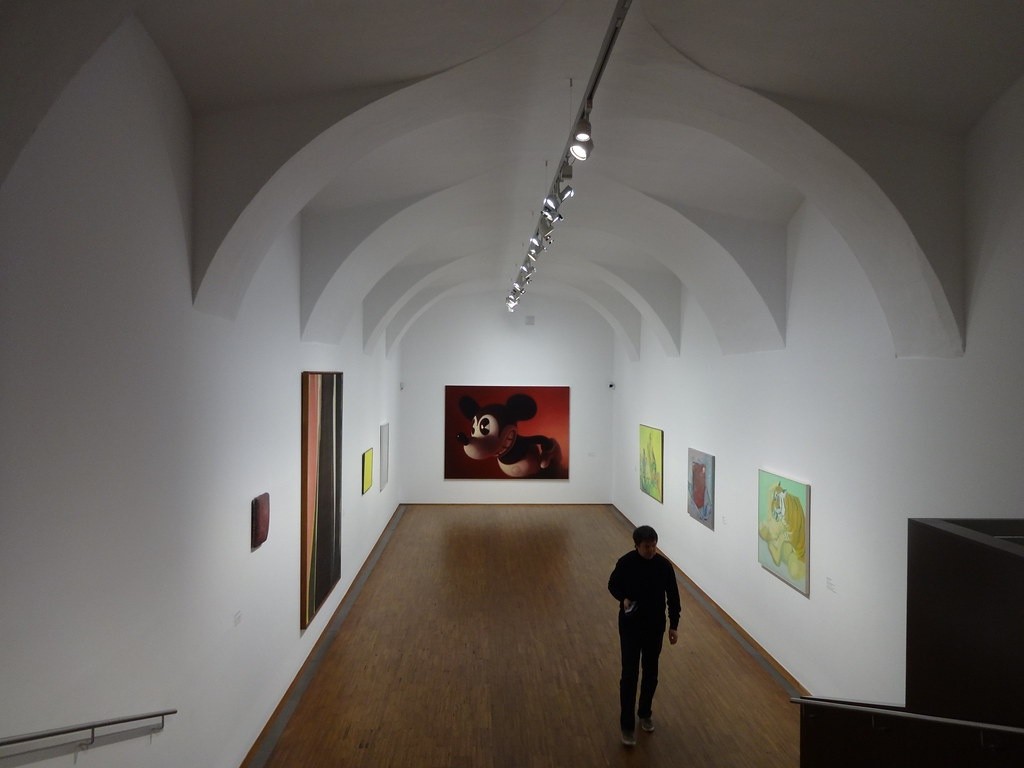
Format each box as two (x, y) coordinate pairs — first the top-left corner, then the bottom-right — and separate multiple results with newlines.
(621, 732), (636, 745)
(639, 717), (654, 732)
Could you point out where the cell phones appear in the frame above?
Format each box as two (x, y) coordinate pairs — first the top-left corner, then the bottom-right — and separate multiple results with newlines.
(625, 602), (635, 613)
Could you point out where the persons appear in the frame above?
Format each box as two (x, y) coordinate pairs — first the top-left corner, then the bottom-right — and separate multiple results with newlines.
(608, 525), (681, 748)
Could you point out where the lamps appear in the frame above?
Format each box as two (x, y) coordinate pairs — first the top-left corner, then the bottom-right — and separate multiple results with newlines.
(505, 164), (572, 313)
(570, 139), (594, 161)
(575, 119), (591, 142)
